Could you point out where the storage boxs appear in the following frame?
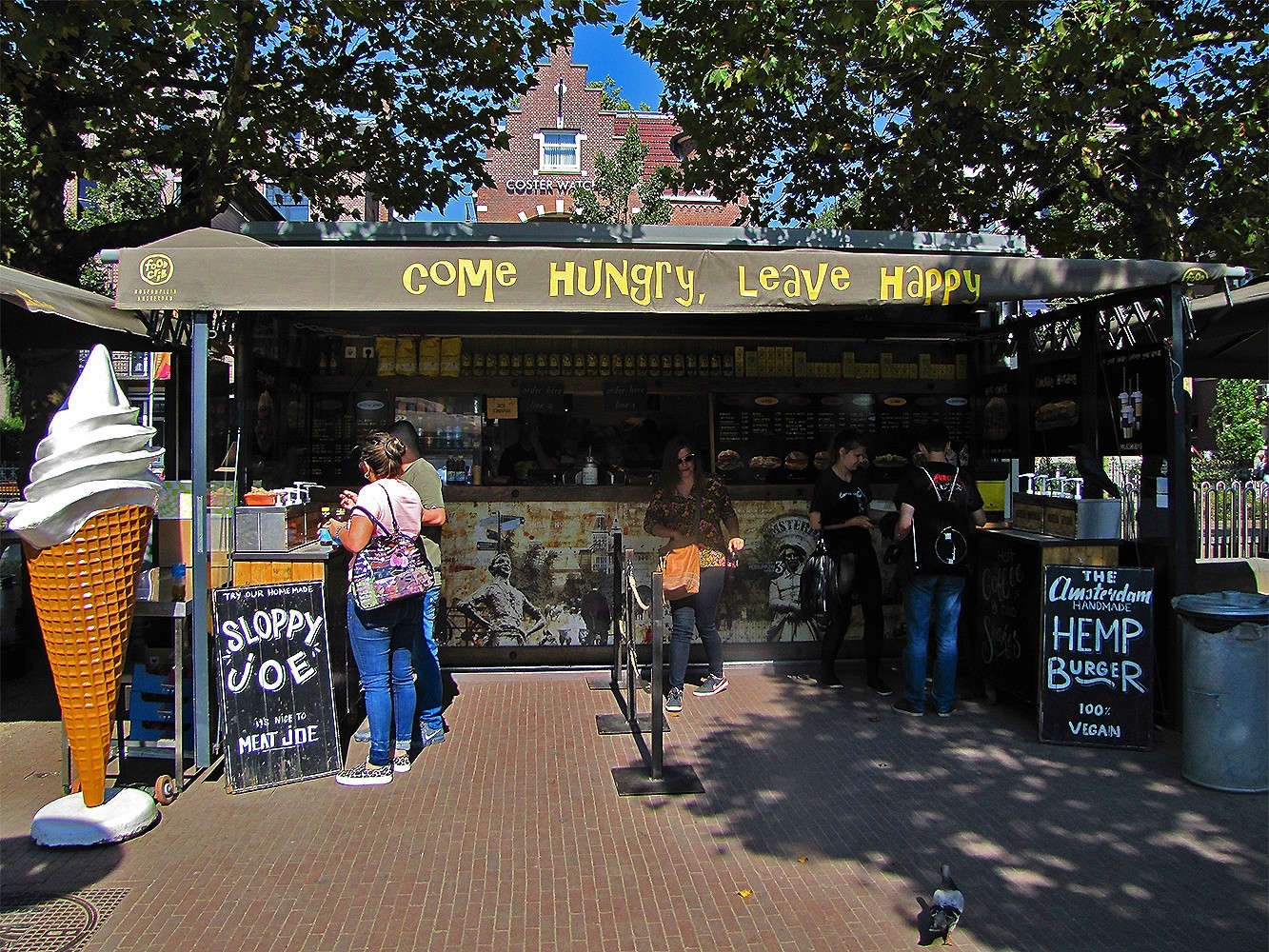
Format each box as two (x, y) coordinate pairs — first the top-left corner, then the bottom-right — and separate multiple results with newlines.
(158, 519), (190, 569)
(128, 617), (193, 752)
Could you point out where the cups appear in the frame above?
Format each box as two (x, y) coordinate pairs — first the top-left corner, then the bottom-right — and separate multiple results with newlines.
(171, 562), (187, 601)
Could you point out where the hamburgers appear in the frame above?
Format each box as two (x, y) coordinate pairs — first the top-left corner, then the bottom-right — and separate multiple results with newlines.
(716, 450), (744, 472)
(784, 451), (808, 470)
(814, 451), (830, 471)
(1034, 400), (1078, 429)
(985, 397), (1011, 438)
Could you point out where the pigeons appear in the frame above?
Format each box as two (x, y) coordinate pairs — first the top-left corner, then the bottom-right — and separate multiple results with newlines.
(928, 863), (965, 946)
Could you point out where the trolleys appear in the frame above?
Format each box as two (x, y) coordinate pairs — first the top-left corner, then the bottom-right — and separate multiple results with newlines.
(53, 599), (194, 805)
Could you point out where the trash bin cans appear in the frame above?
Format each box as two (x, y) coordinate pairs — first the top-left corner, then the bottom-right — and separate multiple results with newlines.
(1170, 590), (1269, 794)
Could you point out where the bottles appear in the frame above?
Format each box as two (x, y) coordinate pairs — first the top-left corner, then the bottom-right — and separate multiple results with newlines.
(399, 395), (480, 484)
(320, 505), (334, 545)
(331, 509), (351, 549)
(582, 454), (599, 485)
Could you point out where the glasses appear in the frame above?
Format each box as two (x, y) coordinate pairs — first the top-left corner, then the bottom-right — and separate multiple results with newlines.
(675, 454), (698, 464)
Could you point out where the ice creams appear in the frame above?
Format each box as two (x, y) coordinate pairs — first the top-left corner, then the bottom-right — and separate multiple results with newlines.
(6, 343), (165, 806)
(957, 445), (969, 466)
(946, 449), (956, 467)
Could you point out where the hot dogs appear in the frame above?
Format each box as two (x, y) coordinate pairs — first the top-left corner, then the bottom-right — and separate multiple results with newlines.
(750, 456), (781, 469)
(873, 455), (907, 468)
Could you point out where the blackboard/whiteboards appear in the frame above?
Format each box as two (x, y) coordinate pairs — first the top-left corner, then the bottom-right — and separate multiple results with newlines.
(212, 579), (343, 795)
(1038, 562), (1157, 750)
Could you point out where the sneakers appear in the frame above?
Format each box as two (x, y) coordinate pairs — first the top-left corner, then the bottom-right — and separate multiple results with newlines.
(665, 687), (684, 711)
(366, 755), (411, 773)
(336, 762), (393, 785)
(355, 728), (372, 743)
(410, 724), (448, 748)
(891, 699), (926, 716)
(693, 670), (729, 697)
(934, 700), (957, 717)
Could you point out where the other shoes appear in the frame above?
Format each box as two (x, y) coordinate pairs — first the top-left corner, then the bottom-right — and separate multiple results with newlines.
(819, 646), (845, 689)
(864, 647), (893, 695)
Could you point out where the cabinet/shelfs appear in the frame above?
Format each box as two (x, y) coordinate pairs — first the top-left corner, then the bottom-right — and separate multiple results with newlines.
(965, 525), (1121, 731)
(389, 389), (483, 486)
(226, 538), (364, 726)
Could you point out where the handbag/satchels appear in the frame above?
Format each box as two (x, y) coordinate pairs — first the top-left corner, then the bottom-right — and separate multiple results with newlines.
(657, 535), (701, 600)
(349, 483), (435, 612)
(801, 524), (838, 626)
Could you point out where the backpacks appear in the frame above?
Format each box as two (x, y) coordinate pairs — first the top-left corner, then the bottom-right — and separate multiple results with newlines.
(910, 465), (971, 573)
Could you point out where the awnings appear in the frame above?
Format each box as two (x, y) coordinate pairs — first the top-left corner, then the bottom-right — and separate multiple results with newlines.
(117, 227), (1229, 318)
(0, 264), (235, 358)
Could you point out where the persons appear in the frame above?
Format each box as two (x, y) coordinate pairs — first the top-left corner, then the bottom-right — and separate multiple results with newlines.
(591, 440), (638, 488)
(890, 419), (989, 718)
(1253, 448), (1269, 505)
(766, 544), (829, 643)
(316, 430), (422, 788)
(497, 409), (568, 486)
(355, 420), (447, 753)
(809, 430), (895, 698)
(458, 551), (549, 649)
(644, 436), (747, 713)
(529, 579), (740, 648)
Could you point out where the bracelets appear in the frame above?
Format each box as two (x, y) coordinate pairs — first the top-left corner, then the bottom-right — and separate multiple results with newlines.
(336, 526), (347, 539)
